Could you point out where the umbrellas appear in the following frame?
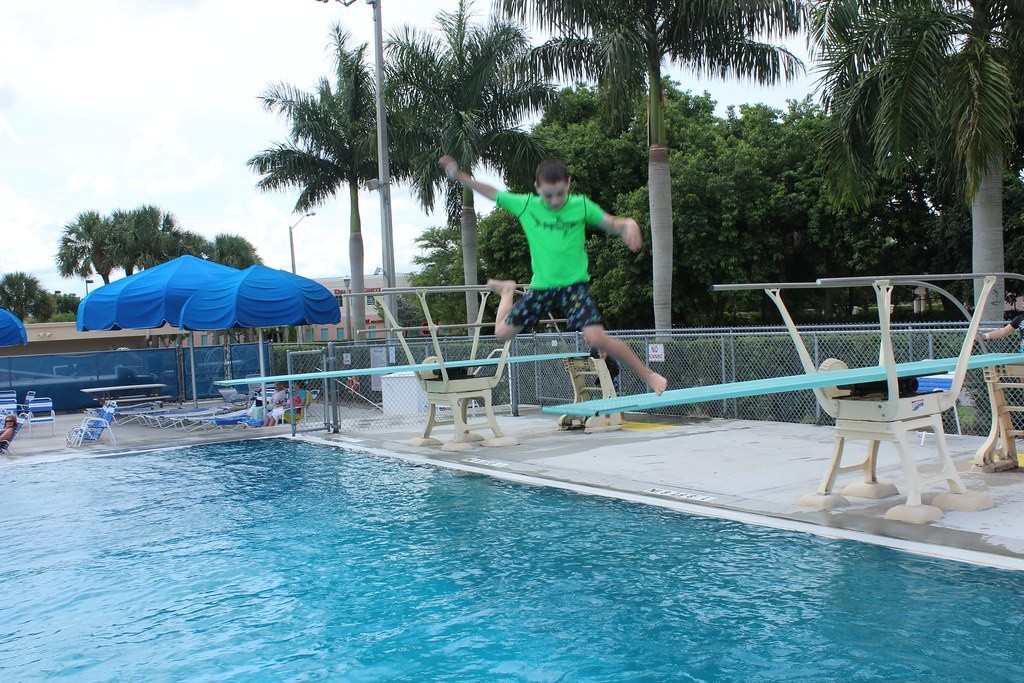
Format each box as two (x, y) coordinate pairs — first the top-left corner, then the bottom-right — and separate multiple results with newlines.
(76, 255), (242, 412)
(179, 263), (342, 417)
(0, 308), (28, 347)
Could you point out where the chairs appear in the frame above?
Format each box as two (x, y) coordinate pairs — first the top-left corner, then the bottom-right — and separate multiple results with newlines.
(0, 412), (30, 456)
(87, 399), (230, 432)
(19, 392), (35, 418)
(66, 400), (110, 445)
(236, 389), (323, 432)
(208, 392), (278, 432)
(73, 402), (118, 448)
(0, 410), (14, 430)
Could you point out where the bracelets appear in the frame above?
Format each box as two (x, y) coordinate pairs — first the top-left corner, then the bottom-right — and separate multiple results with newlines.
(984, 333), (990, 340)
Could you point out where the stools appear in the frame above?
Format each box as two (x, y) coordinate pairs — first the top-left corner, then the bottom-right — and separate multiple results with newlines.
(794, 415), (995, 524)
(765, 275), (996, 420)
(374, 290), (514, 393)
(405, 389), (519, 451)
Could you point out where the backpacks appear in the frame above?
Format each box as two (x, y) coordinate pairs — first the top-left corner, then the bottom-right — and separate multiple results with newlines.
(289, 396), (301, 414)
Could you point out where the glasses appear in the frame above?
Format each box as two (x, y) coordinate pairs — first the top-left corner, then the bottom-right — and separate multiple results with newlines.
(5, 420), (14, 422)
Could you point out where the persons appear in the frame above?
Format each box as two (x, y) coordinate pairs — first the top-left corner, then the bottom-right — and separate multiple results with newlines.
(0, 415), (18, 449)
(264, 380), (308, 427)
(248, 381), (286, 416)
(974, 297), (1024, 387)
(440, 155), (668, 396)
